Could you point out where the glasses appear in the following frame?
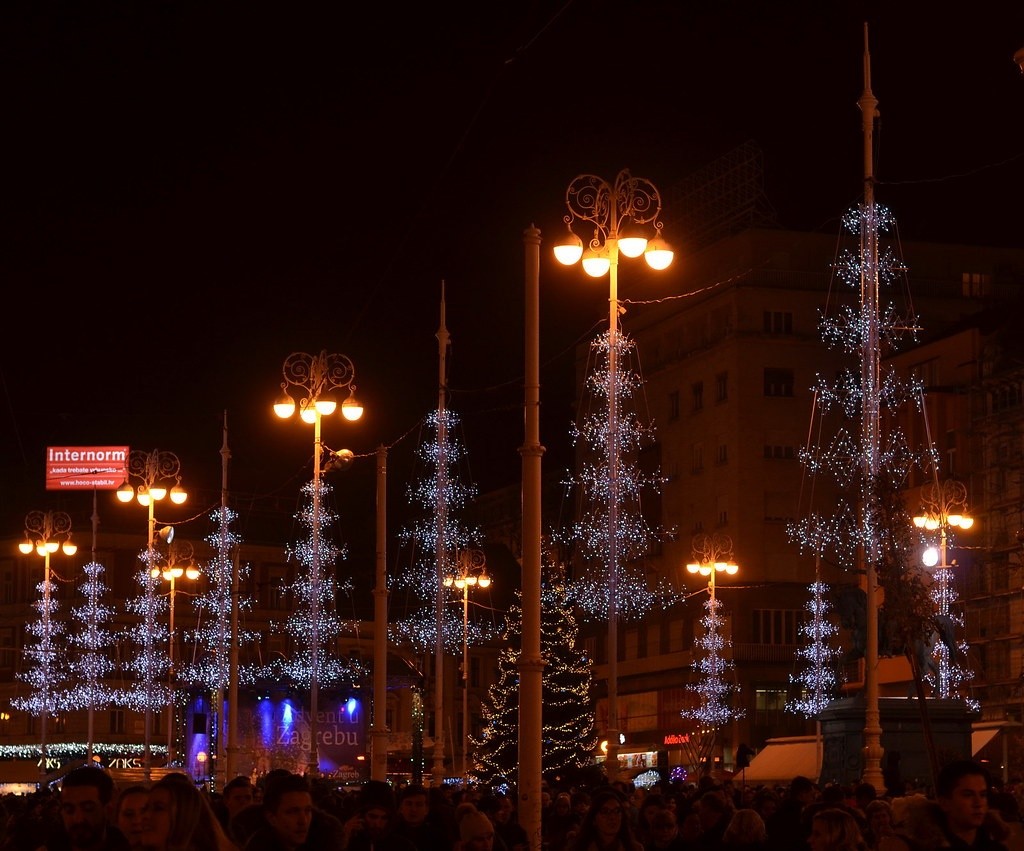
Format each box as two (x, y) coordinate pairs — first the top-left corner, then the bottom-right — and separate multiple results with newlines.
(596, 805), (623, 816)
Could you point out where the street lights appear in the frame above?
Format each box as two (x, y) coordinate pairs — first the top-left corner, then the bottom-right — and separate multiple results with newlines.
(549, 164), (673, 782)
(441, 532), (491, 786)
(682, 509), (740, 790)
(909, 477), (975, 703)
(115, 441), (189, 785)
(18, 498), (77, 794)
(271, 336), (362, 782)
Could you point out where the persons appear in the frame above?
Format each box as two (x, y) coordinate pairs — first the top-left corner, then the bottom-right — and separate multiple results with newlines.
(0, 753), (1023, 851)
